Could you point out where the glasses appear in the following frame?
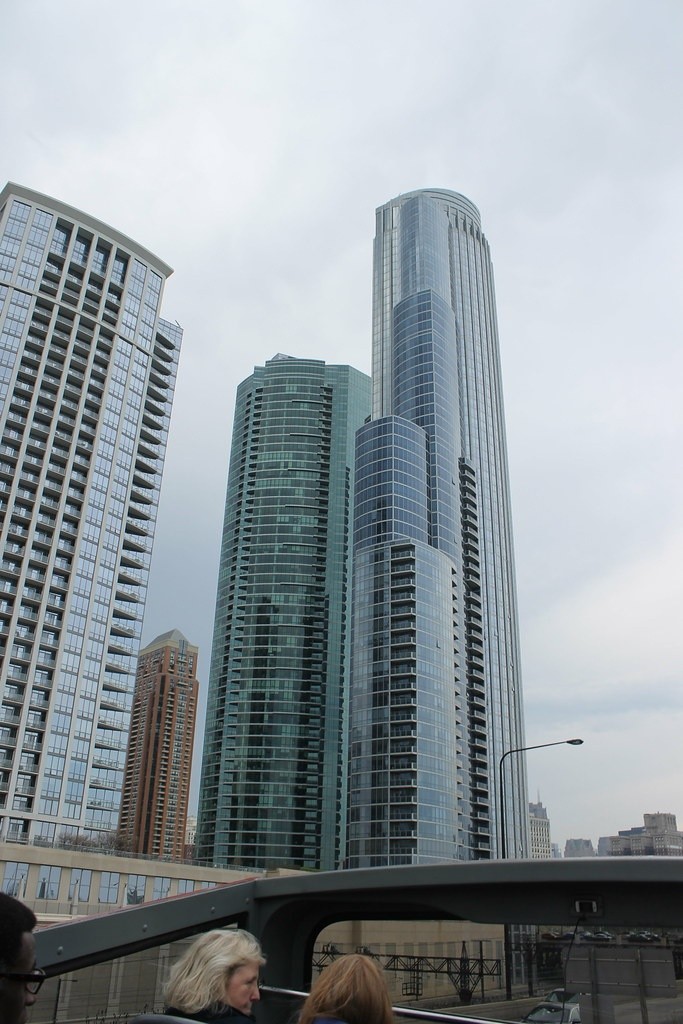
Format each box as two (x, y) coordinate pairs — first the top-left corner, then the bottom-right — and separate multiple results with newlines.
(0, 969), (47, 995)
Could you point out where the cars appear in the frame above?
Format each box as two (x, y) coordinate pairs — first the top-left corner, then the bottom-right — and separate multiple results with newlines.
(541, 931), (662, 943)
(541, 987), (590, 1003)
(520, 1002), (582, 1024)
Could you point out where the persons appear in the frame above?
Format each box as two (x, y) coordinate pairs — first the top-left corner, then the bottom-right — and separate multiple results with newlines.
(0, 890), (47, 1024)
(296, 954), (396, 1024)
(160, 927), (267, 1023)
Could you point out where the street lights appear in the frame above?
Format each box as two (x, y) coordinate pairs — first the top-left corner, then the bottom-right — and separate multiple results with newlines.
(500, 738), (585, 1001)
